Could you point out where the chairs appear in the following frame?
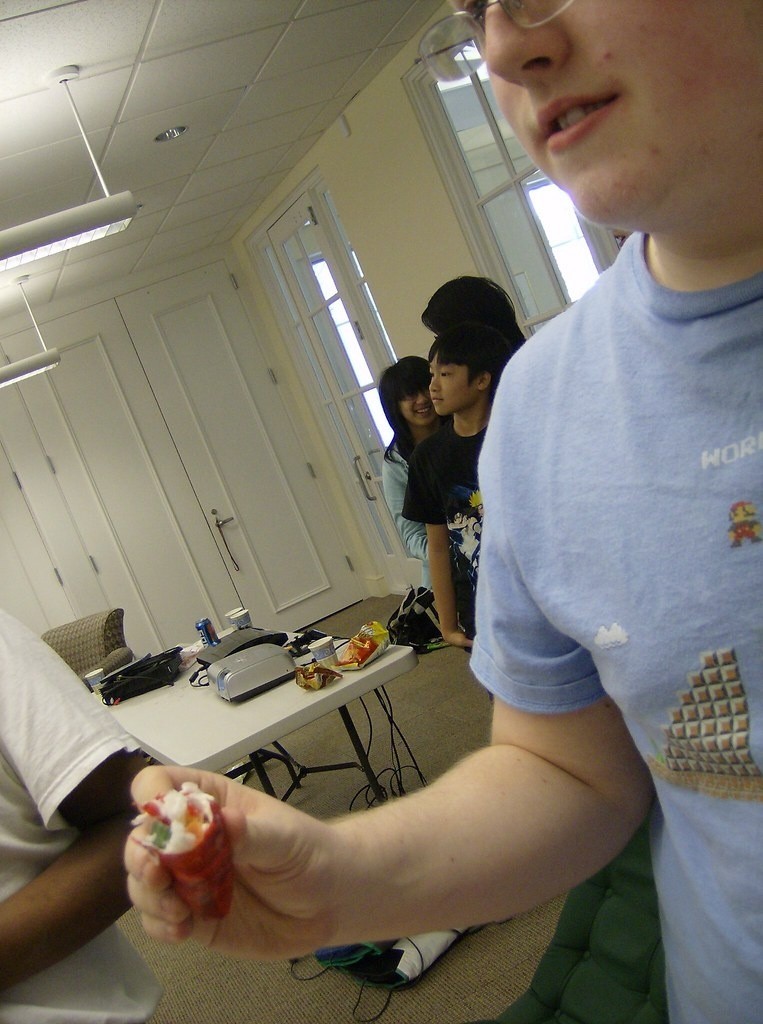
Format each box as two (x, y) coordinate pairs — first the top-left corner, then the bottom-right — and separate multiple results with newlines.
(481, 826), (670, 1024)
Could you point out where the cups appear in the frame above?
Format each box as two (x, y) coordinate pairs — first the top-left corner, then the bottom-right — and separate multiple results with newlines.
(225, 607), (244, 630)
(85, 668), (105, 701)
(231, 609), (252, 630)
(308, 636), (338, 670)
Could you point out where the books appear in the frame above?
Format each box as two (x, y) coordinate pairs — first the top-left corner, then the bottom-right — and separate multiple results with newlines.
(99, 652), (151, 684)
(128, 645), (183, 672)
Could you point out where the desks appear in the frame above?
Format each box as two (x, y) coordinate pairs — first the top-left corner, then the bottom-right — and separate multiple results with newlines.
(89, 626), (420, 807)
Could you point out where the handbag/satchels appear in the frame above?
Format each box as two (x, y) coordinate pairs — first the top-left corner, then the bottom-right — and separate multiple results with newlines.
(387, 584), (443, 649)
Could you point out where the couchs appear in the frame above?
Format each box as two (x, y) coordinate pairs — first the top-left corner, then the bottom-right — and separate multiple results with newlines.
(40, 608), (133, 694)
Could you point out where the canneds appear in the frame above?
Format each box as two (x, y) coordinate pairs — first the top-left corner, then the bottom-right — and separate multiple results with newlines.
(194, 617), (219, 648)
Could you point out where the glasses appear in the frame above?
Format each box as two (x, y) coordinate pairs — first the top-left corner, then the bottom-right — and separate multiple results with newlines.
(411, 0), (572, 82)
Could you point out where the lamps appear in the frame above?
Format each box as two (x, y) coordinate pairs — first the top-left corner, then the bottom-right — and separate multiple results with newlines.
(0, 66), (137, 260)
(0, 274), (61, 388)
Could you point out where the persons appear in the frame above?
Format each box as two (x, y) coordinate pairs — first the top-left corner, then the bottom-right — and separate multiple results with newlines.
(0, 607), (152, 1024)
(411, 320), (514, 651)
(124, 0), (762, 1021)
(377, 355), (447, 590)
